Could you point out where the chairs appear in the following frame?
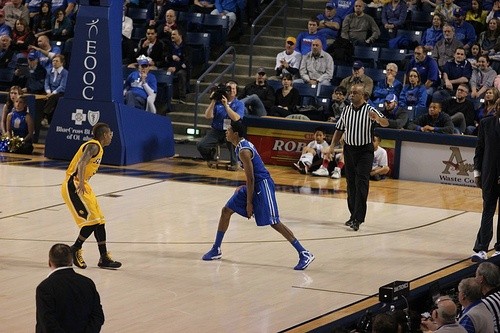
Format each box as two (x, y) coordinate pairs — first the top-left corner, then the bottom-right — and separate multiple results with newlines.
(0, 0), (433, 120)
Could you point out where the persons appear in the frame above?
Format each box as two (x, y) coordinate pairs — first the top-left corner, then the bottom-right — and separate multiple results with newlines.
(197, 81), (245, 171)
(0, 0), (500, 180)
(203, 122), (314, 270)
(325, 84), (389, 231)
(61, 123), (121, 271)
(472, 98), (500, 262)
(36, 244), (105, 333)
(330, 261), (500, 333)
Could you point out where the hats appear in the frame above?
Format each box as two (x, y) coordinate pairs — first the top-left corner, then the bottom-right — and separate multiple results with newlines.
(352, 61), (366, 69)
(27, 51), (39, 60)
(138, 59), (149, 66)
(286, 36), (296, 44)
(453, 8), (463, 16)
(384, 94), (397, 102)
(326, 1), (335, 9)
(257, 67), (266, 74)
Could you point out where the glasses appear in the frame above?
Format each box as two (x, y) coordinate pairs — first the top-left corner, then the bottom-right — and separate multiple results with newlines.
(409, 75), (418, 77)
(457, 89), (468, 93)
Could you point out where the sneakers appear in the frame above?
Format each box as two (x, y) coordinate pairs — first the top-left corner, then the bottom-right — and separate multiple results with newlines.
(294, 250), (315, 270)
(202, 245), (222, 260)
(311, 165), (329, 176)
(471, 250), (488, 262)
(97, 255), (122, 268)
(70, 245), (87, 269)
(491, 251), (500, 257)
(331, 167), (341, 179)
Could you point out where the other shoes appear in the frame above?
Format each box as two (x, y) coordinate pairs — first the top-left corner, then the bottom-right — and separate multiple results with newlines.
(207, 150), (217, 168)
(292, 163), (302, 172)
(352, 220), (360, 231)
(371, 174), (381, 180)
(229, 161), (241, 171)
(345, 219), (354, 226)
(298, 161), (308, 174)
(40, 118), (51, 128)
